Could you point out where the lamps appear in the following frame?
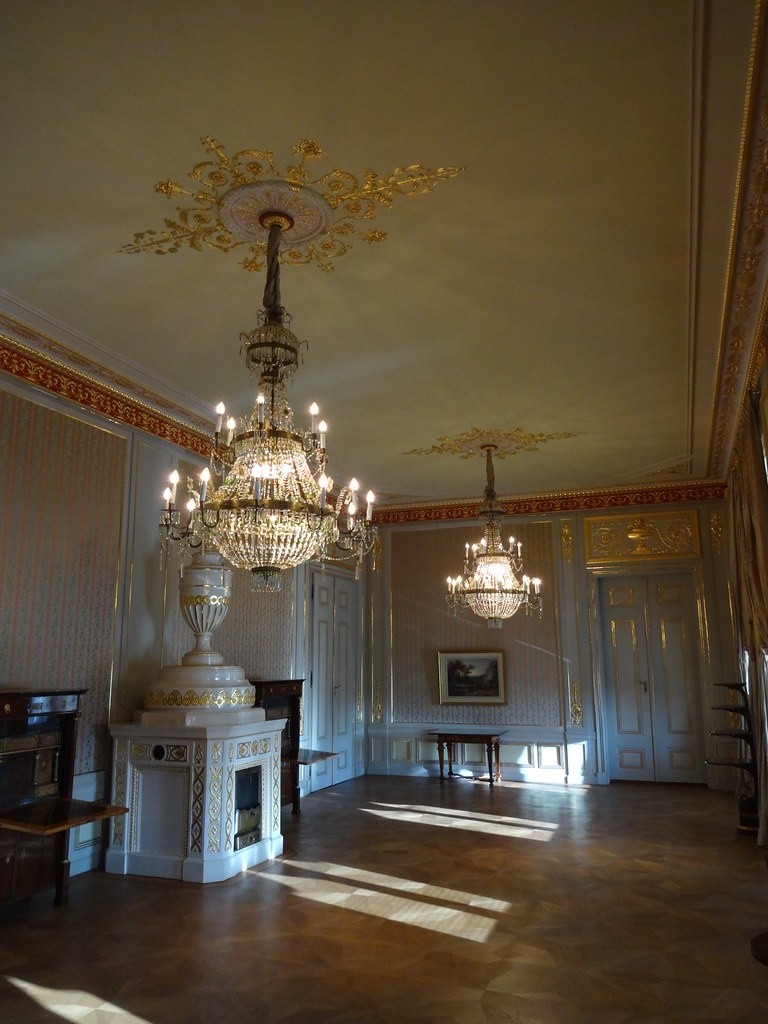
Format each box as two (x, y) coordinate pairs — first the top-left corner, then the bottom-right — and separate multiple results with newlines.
(160, 306), (382, 598)
(443, 502), (544, 632)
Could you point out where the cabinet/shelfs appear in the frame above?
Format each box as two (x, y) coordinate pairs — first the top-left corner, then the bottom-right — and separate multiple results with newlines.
(247, 680), (337, 817)
(0, 688), (130, 908)
(704, 682), (761, 833)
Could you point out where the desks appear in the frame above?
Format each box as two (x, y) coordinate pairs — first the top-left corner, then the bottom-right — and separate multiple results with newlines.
(428, 728), (509, 784)
